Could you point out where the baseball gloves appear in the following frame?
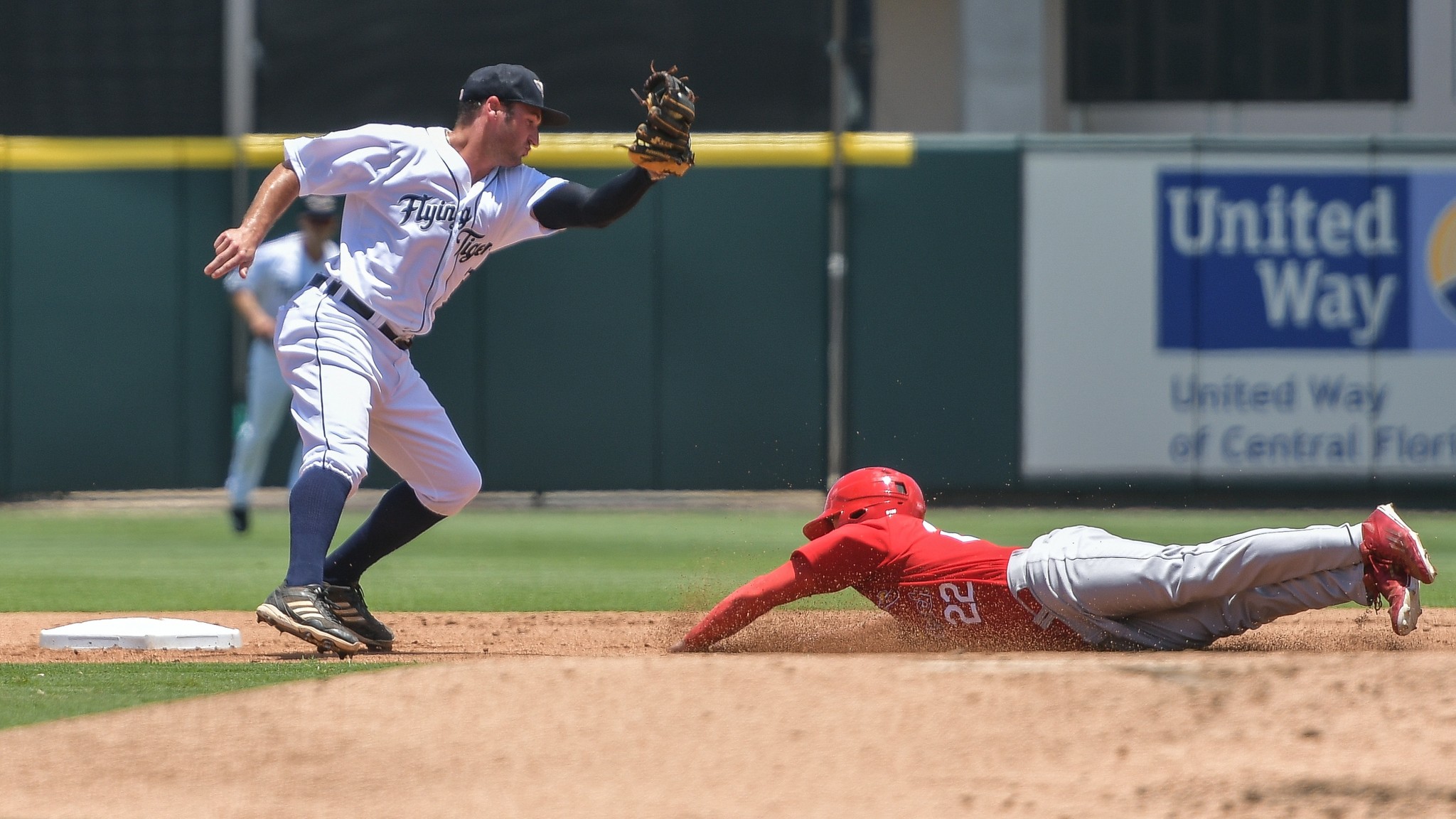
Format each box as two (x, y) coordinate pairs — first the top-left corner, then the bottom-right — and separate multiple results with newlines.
(628, 71), (696, 177)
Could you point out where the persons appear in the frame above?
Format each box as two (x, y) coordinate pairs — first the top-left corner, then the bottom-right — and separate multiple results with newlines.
(669, 465), (1438, 657)
(221, 197), (337, 532)
(204, 61), (697, 658)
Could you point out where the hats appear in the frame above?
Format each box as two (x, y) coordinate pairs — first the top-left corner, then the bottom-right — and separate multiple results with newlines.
(458, 63), (570, 124)
(304, 195), (341, 221)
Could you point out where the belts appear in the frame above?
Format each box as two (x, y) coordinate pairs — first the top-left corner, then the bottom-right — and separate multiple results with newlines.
(1019, 589), (1087, 646)
(309, 275), (412, 349)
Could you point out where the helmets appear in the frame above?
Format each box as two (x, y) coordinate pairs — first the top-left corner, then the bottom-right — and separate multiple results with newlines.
(803, 466), (927, 540)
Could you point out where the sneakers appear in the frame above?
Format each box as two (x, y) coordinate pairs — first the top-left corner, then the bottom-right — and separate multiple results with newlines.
(1363, 503), (1437, 585)
(1365, 568), (1423, 636)
(322, 583), (395, 652)
(255, 580), (368, 660)
